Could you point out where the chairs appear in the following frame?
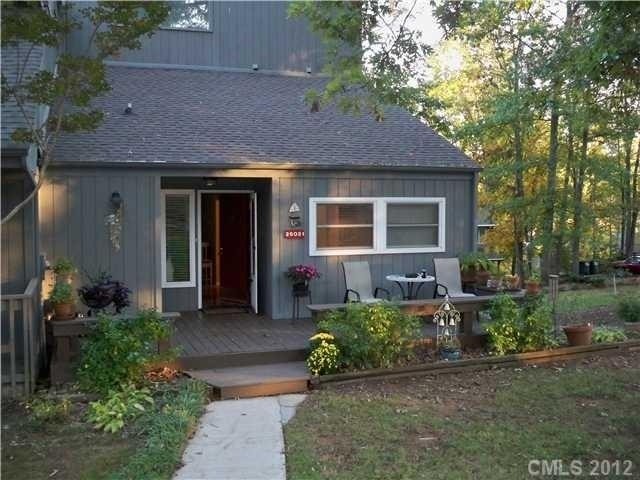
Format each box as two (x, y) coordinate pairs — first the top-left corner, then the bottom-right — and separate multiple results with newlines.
(342, 261), (390, 303)
(432, 257), (479, 322)
(201, 242), (213, 288)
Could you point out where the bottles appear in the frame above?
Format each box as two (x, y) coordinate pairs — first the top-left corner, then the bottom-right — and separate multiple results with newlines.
(420, 269), (428, 279)
(487, 279), (498, 289)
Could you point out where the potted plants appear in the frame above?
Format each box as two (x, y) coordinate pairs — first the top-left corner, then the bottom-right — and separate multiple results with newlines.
(49, 258), (77, 284)
(438, 337), (461, 358)
(525, 270), (540, 292)
(460, 252), (495, 282)
(48, 284), (76, 320)
(77, 266), (132, 317)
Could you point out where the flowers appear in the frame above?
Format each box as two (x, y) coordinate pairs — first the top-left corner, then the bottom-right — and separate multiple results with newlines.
(285, 264), (320, 283)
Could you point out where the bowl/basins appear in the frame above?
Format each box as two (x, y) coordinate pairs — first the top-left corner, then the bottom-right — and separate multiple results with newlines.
(564, 325), (596, 344)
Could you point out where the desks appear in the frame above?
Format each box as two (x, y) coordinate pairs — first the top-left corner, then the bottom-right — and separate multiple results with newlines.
(386, 274), (435, 301)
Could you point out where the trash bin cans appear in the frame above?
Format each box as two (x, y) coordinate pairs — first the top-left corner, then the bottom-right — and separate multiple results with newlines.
(579, 260), (600, 276)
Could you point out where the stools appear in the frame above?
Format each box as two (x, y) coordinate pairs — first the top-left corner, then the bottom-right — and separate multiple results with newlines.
(292, 291), (312, 324)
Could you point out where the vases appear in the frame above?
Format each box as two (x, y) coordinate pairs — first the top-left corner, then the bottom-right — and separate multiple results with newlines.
(294, 283), (309, 291)
(563, 322), (593, 345)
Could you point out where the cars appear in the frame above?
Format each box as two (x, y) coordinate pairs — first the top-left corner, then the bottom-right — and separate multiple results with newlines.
(611, 256), (639, 275)
